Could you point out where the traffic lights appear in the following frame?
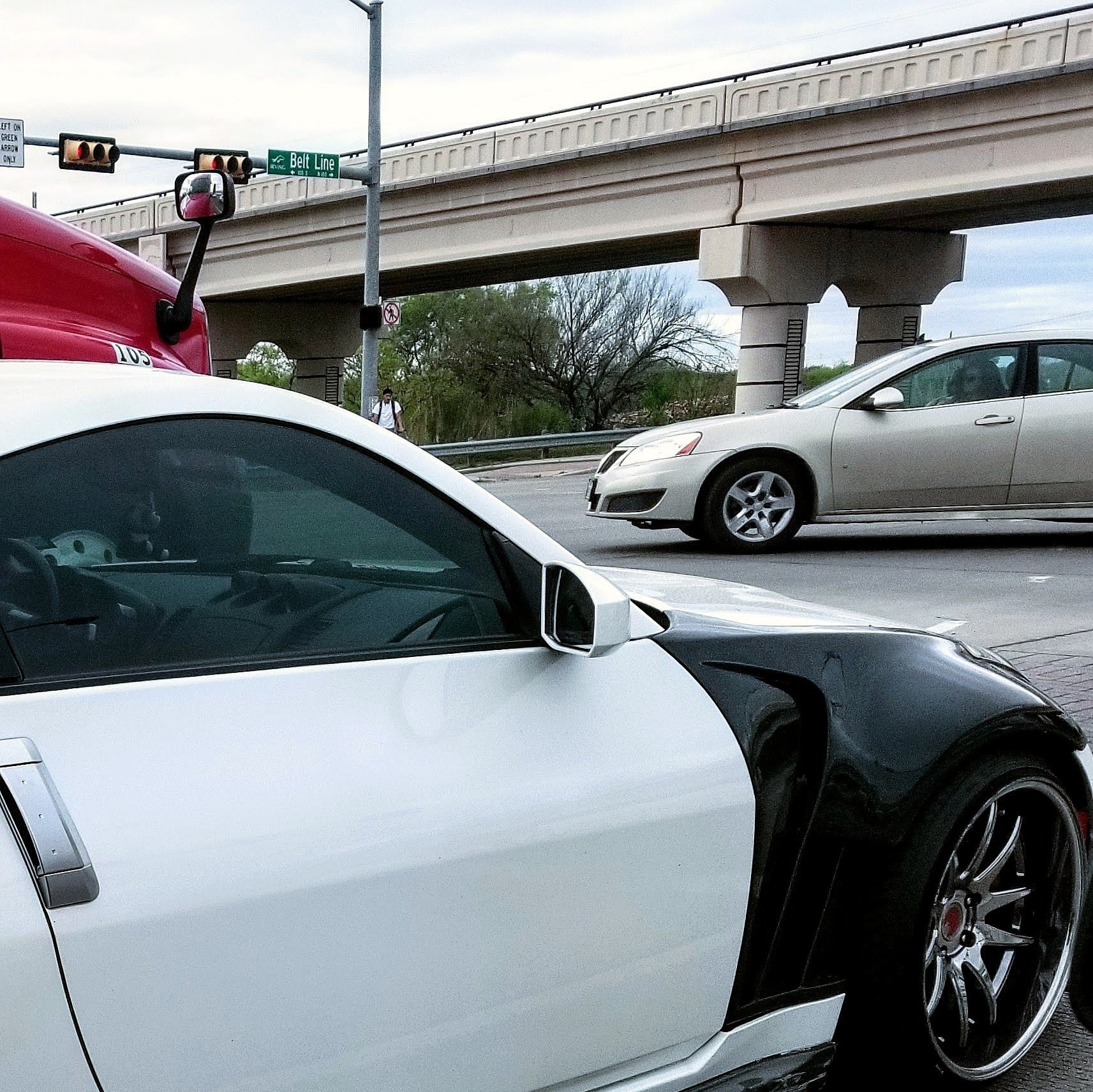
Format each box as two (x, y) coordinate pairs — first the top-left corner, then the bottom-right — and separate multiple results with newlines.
(194, 148), (254, 185)
(58, 132), (120, 173)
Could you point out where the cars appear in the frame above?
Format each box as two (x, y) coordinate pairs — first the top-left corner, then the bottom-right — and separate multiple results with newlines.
(0, 357), (1093, 1091)
(211, 191), (224, 203)
(583, 328), (1093, 554)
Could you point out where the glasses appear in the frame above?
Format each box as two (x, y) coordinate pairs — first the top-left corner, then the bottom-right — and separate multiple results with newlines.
(384, 395), (391, 397)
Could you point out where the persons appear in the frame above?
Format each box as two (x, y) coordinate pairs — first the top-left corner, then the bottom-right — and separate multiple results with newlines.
(925, 361), (1004, 407)
(370, 388), (404, 436)
